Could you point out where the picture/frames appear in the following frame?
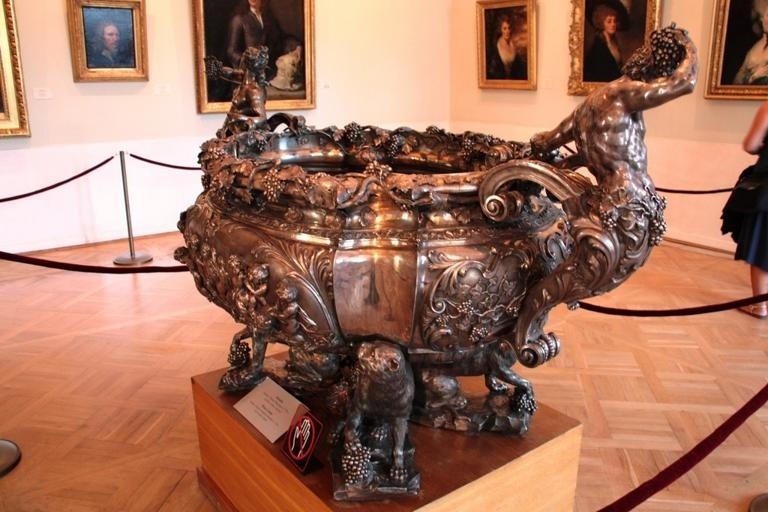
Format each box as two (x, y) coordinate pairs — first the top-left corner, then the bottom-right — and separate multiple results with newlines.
(567, 0), (663, 96)
(703, 0), (768, 101)
(65, 0), (150, 83)
(0, 0), (31, 138)
(476, 0), (537, 91)
(192, 0), (317, 114)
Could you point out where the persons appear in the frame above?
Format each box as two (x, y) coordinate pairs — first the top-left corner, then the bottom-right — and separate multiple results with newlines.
(225, 0), (305, 98)
(486, 14), (527, 78)
(88, 21), (135, 67)
(729, 0), (768, 85)
(719, 103), (768, 318)
(581, 4), (624, 82)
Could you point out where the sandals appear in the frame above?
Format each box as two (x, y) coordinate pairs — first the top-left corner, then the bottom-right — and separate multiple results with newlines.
(739, 305), (768, 318)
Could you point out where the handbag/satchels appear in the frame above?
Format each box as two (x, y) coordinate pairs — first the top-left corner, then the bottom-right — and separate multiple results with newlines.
(729, 176), (768, 213)
